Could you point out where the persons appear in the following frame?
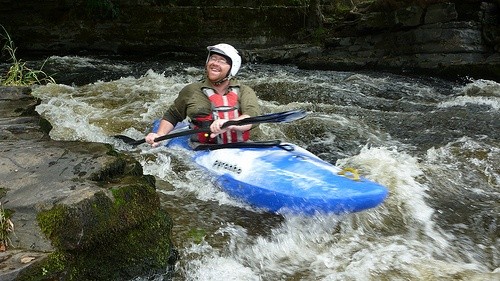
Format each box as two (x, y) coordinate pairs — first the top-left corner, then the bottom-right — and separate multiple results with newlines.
(144, 43), (261, 150)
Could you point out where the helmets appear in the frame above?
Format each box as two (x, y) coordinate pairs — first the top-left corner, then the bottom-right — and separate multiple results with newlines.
(207, 43), (241, 85)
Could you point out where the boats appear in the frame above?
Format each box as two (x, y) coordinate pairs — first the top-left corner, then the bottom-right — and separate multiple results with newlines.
(151, 116), (387, 215)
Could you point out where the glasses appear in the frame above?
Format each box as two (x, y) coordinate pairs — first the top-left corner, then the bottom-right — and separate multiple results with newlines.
(208, 57), (226, 63)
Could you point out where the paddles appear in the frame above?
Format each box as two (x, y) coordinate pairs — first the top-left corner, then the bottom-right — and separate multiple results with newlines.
(108, 108), (308, 149)
